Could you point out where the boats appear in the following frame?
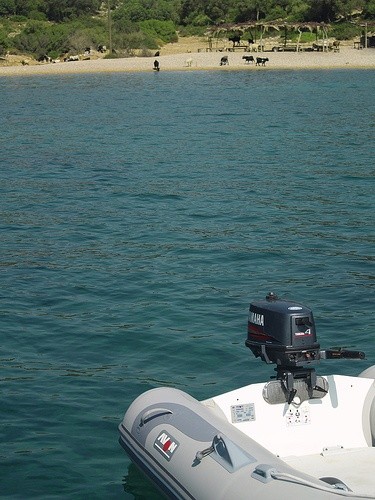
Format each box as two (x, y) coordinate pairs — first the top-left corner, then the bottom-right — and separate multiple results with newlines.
(117, 290), (375, 500)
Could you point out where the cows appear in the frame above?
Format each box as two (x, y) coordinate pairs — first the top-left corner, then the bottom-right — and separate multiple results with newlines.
(183, 57), (193, 67)
(228, 36), (241, 46)
(37, 43), (108, 65)
(256, 57), (269, 67)
(152, 59), (160, 72)
(245, 43), (259, 53)
(219, 55), (229, 66)
(154, 50), (159, 56)
(242, 55), (255, 64)
